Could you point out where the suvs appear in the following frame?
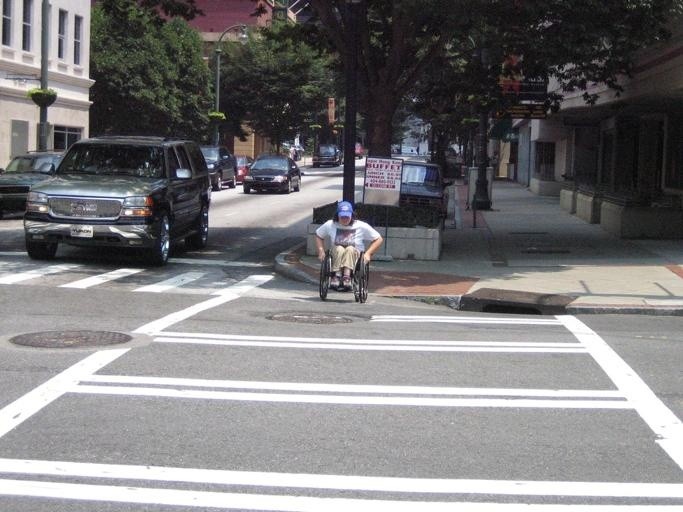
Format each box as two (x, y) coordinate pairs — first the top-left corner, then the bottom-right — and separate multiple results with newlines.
(18, 136), (212, 267)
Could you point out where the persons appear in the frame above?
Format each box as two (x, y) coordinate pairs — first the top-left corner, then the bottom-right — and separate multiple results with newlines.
(314, 201), (385, 287)
(490, 151), (500, 178)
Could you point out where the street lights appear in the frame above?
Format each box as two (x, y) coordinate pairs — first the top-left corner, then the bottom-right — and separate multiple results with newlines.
(472, 39), (513, 210)
(213, 23), (249, 145)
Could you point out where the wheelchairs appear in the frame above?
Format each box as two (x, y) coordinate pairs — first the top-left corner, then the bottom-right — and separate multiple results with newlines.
(320, 248), (370, 303)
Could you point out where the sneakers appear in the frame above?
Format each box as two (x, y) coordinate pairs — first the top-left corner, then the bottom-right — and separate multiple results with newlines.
(341, 276), (352, 289)
(331, 275), (342, 288)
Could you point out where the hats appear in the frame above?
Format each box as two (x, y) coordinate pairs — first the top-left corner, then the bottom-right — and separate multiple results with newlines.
(337, 201), (353, 219)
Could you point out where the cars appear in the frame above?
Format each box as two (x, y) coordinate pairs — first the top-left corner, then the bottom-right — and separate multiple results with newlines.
(199, 147), (238, 189)
(242, 153), (303, 194)
(235, 155), (256, 181)
(398, 162), (452, 218)
(0, 152), (64, 219)
(312, 142), (363, 168)
